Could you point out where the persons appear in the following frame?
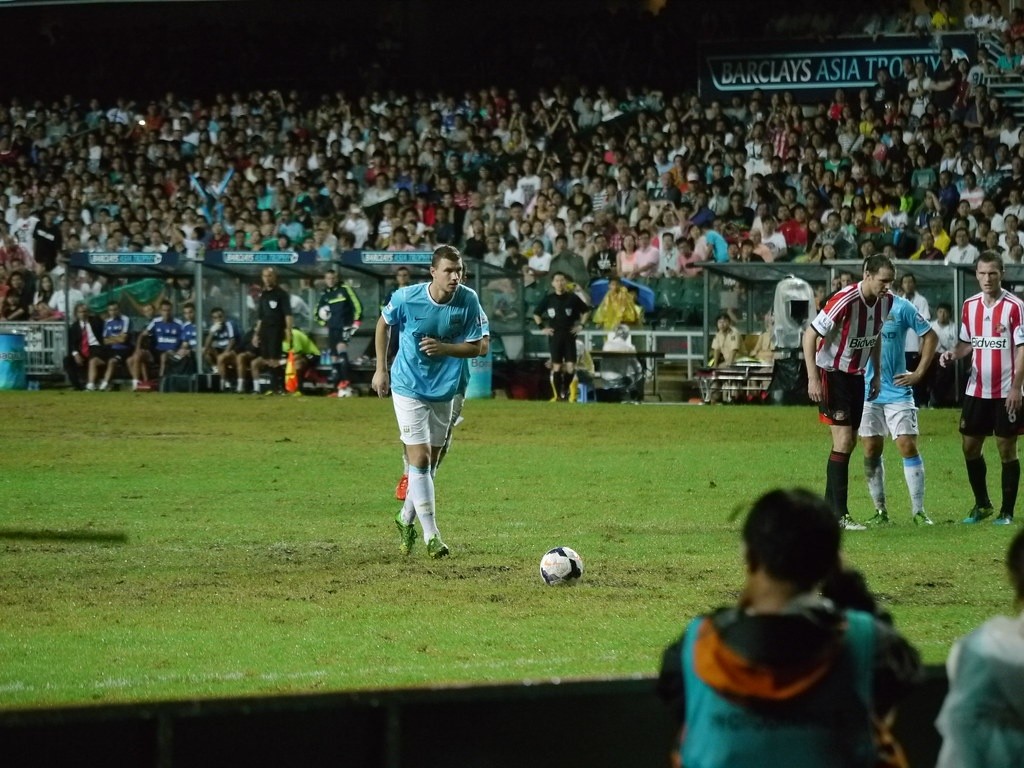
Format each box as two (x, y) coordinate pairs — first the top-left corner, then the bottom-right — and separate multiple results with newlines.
(651, 487), (923, 768)
(940, 251), (1024, 525)
(372, 247), (483, 558)
(395, 264), (489, 499)
(0, 0), (1024, 403)
(859, 258), (939, 527)
(802, 254), (897, 529)
(936, 529), (1024, 768)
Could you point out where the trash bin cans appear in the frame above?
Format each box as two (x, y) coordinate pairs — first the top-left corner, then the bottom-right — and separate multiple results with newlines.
(0, 333), (27, 390)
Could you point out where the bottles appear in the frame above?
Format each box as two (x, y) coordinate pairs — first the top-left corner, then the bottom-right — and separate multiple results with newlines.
(320, 349), (331, 365)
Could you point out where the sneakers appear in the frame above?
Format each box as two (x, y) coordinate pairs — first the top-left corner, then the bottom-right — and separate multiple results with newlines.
(395, 474), (408, 500)
(395, 511), (423, 554)
(426, 535), (449, 558)
(867, 511), (889, 525)
(912, 510), (936, 527)
(994, 511), (1013, 526)
(838, 514), (866, 530)
(964, 503), (994, 523)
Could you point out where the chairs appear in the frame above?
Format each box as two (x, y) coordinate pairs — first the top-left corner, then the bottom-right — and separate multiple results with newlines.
(159, 346), (776, 406)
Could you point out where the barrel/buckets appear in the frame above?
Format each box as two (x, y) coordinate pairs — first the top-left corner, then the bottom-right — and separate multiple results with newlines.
(0, 326), (28, 389)
(467, 343), (495, 400)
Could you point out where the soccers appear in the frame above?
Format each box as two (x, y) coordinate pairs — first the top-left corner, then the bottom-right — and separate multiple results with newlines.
(539, 547), (584, 587)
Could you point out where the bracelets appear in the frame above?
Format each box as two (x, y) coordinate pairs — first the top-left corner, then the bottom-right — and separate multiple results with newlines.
(538, 323), (544, 330)
(580, 323), (585, 329)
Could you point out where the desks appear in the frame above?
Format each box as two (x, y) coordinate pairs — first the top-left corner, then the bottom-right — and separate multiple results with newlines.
(589, 349), (665, 402)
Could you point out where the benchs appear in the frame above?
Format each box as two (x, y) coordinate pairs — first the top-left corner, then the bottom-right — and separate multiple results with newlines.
(82, 329), (155, 379)
